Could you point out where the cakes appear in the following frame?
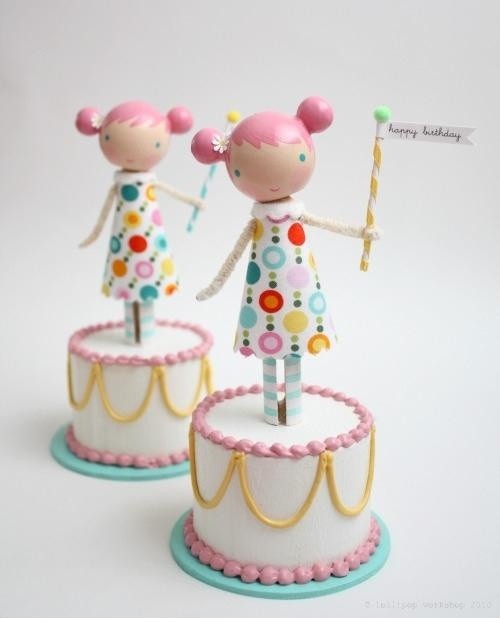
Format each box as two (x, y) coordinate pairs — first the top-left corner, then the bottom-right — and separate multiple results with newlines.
(65, 101), (239, 468)
(182, 96), (390, 586)
